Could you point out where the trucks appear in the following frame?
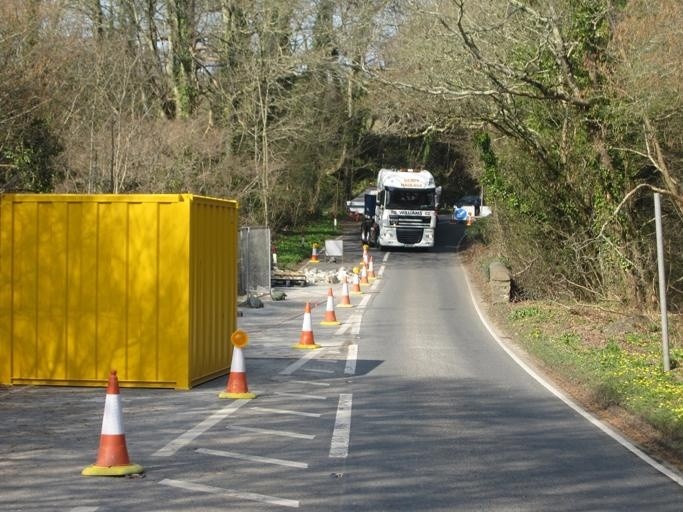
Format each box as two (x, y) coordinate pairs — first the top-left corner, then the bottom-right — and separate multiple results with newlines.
(359, 167), (442, 251)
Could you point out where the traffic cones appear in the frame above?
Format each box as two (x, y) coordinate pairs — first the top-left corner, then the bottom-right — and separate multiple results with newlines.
(350, 273), (363, 295)
(358, 265), (371, 287)
(361, 249), (368, 268)
(367, 255), (377, 280)
(76, 365), (146, 479)
(216, 347), (257, 400)
(466, 218), (471, 226)
(318, 286), (342, 326)
(290, 299), (322, 349)
(308, 242), (320, 263)
(335, 275), (354, 309)
